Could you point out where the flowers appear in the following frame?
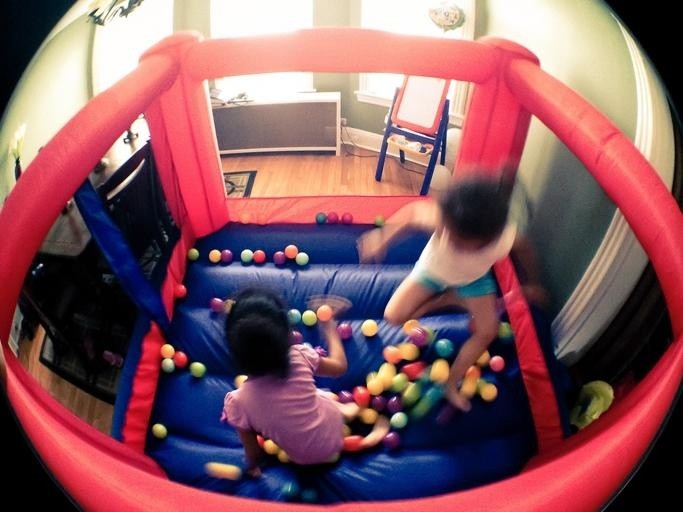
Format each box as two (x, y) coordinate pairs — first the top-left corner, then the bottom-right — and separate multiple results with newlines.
(6, 123), (26, 161)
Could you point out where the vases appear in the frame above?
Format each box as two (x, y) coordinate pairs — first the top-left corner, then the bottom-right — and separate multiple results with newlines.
(14, 155), (23, 181)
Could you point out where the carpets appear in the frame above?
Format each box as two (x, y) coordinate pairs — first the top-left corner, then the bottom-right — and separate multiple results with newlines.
(37, 168), (257, 406)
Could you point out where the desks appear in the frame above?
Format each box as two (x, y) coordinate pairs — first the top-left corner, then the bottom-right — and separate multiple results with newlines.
(17, 117), (175, 377)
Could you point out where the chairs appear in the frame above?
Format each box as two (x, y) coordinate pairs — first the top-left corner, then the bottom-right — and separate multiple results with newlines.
(91, 138), (178, 303)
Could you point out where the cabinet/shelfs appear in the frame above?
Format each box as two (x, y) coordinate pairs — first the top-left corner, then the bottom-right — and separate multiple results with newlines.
(214, 92), (341, 157)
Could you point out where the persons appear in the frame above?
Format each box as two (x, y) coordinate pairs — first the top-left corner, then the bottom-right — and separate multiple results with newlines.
(356, 164), (531, 411)
